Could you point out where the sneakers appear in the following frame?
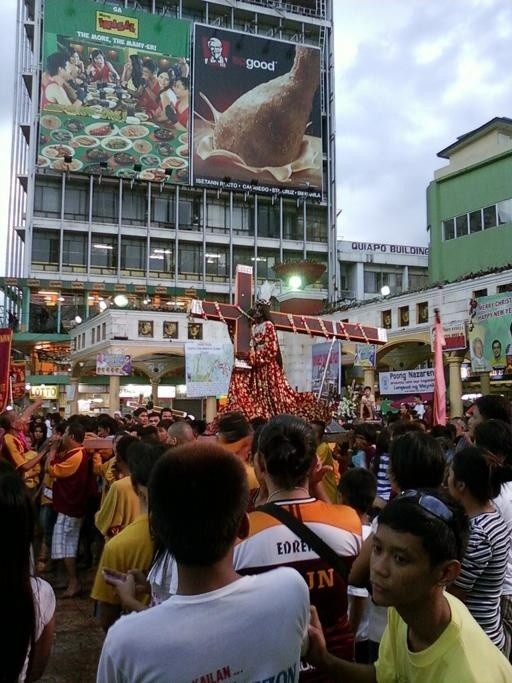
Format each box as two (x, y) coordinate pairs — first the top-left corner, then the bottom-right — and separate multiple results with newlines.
(63, 583), (81, 598)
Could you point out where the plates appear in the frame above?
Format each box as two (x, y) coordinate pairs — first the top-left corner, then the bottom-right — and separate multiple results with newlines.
(40, 81), (189, 180)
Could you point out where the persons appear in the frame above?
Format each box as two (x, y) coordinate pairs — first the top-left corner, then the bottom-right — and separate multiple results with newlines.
(205, 304), (333, 432)
(472, 337), (506, 371)
(41, 50), (188, 131)
(204, 36), (228, 68)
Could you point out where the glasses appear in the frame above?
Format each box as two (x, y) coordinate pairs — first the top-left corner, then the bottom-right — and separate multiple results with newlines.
(396, 490), (453, 521)
